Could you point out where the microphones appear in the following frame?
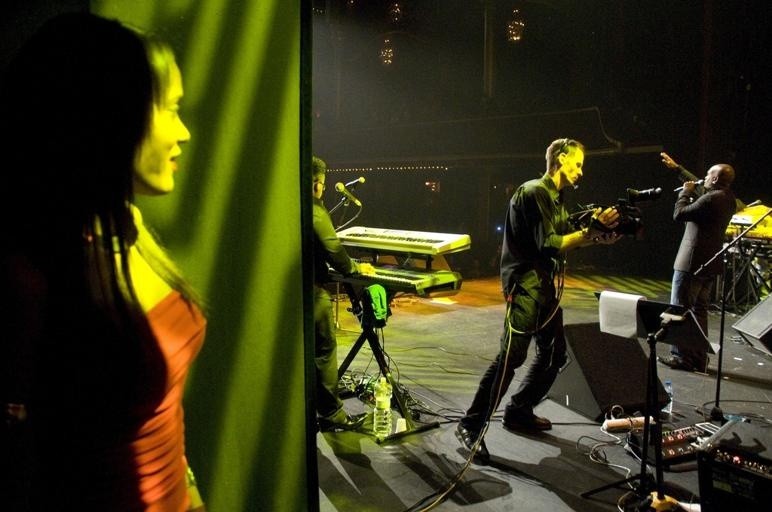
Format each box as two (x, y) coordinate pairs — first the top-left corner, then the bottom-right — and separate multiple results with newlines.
(335, 182), (361, 206)
(345, 177), (365, 187)
(674, 179), (704, 191)
(747, 200), (761, 207)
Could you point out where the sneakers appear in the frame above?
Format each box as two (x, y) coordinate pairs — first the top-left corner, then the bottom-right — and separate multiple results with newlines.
(503, 410), (554, 433)
(454, 420), (492, 461)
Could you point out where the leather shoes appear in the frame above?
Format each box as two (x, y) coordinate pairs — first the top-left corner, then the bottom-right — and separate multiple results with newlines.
(319, 413), (368, 434)
(657, 354), (707, 373)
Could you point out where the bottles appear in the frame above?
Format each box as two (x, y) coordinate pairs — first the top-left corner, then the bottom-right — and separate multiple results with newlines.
(373, 377), (392, 435)
(663, 381), (672, 413)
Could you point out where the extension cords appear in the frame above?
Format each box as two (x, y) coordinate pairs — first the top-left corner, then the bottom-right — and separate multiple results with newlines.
(602, 416), (654, 430)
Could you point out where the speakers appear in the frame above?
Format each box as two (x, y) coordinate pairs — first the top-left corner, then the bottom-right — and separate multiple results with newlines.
(545, 321), (671, 420)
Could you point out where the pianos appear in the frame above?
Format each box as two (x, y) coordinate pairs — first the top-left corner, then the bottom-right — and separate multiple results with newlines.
(328, 226), (472, 297)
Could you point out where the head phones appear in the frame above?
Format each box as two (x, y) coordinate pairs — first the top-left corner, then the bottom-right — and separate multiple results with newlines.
(560, 138), (569, 154)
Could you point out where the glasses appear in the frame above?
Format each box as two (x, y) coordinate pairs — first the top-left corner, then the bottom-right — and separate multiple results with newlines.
(318, 180), (327, 190)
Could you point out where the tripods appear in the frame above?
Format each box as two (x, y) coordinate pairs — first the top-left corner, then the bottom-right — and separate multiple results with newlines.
(580, 326), (654, 500)
(694, 210), (772, 426)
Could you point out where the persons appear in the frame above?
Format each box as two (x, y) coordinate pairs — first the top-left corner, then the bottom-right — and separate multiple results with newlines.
(454, 137), (623, 465)
(0, 16), (210, 512)
(312, 154), (377, 433)
(648, 151), (737, 372)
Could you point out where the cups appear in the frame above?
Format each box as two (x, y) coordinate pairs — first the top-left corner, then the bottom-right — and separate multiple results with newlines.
(395, 417), (406, 434)
